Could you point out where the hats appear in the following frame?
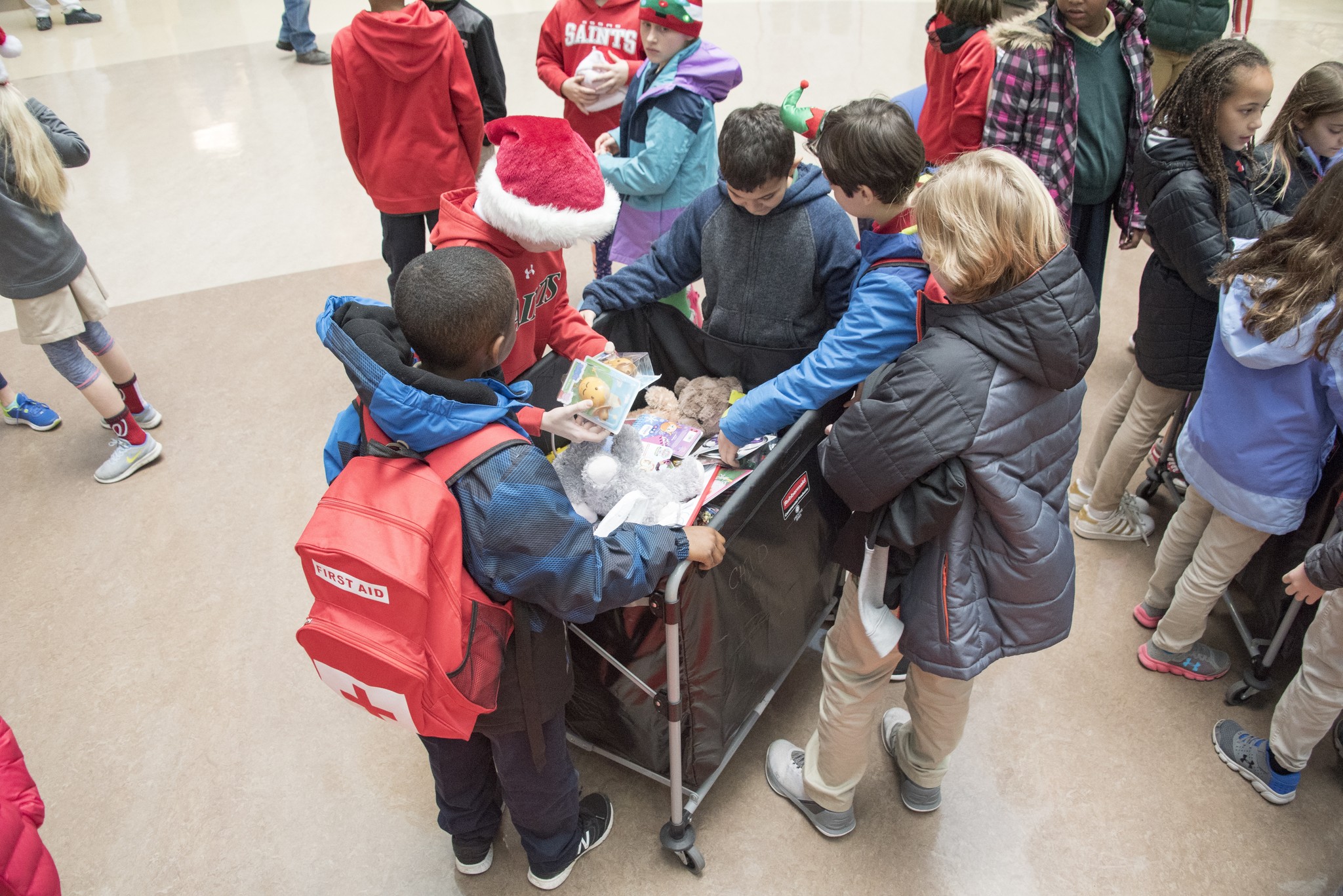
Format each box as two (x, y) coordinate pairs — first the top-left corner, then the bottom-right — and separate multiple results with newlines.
(474, 115), (622, 249)
(638, 0), (703, 37)
(0, 26), (22, 84)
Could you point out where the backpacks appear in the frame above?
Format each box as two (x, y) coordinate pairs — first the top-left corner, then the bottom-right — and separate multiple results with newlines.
(294, 395), (533, 742)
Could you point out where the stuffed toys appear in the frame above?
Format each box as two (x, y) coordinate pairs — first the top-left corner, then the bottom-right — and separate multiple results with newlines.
(641, 369), (744, 452)
(553, 426), (704, 542)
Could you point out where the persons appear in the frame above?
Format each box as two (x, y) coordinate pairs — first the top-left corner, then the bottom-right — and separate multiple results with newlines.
(1, 0), (164, 483)
(1, 722), (65, 895)
(273, 0), (1343, 885)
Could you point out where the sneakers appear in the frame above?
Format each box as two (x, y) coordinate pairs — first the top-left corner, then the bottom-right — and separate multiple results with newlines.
(765, 739), (857, 837)
(881, 707), (942, 812)
(452, 772), (506, 875)
(1138, 636), (1231, 681)
(1, 392), (61, 432)
(100, 404), (163, 429)
(1147, 438), (1188, 494)
(1073, 504), (1155, 546)
(1133, 601), (1168, 628)
(1212, 719), (1300, 804)
(94, 430), (163, 484)
(1068, 478), (1149, 515)
(1334, 719), (1343, 756)
(527, 792), (614, 890)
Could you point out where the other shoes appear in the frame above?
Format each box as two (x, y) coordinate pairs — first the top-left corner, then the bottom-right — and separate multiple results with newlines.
(276, 40), (296, 51)
(889, 658), (911, 681)
(296, 49), (331, 65)
(63, 8), (102, 25)
(36, 17), (52, 31)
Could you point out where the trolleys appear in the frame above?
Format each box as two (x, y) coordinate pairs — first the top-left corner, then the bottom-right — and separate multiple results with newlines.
(502, 301), (910, 879)
